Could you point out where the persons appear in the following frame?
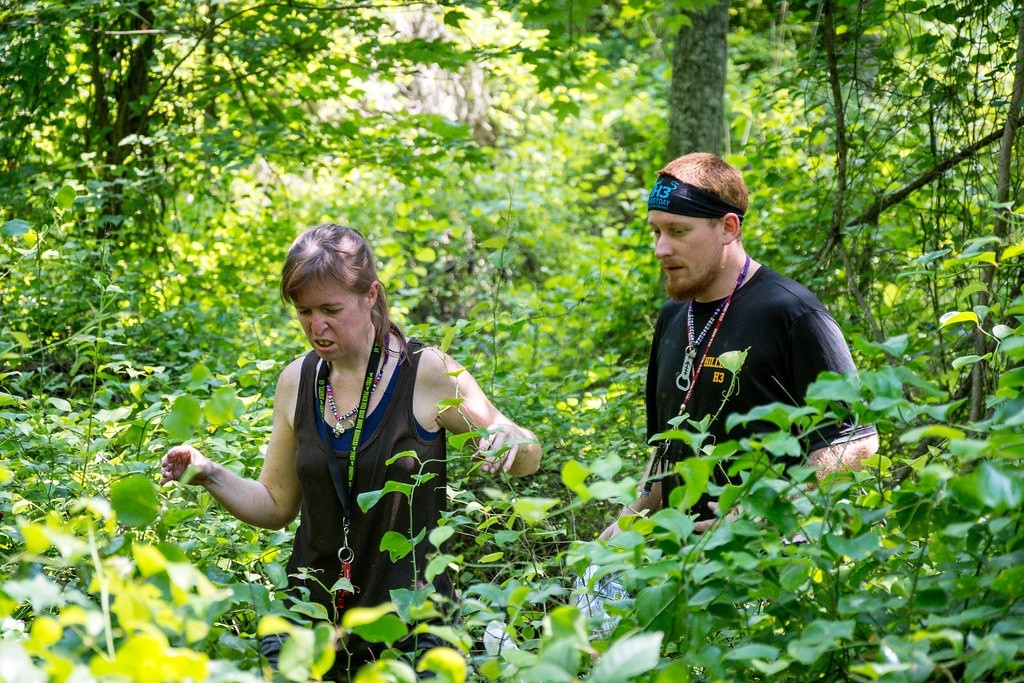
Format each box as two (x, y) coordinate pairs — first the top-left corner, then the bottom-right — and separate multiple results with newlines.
(598, 151), (881, 542)
(157, 223), (542, 679)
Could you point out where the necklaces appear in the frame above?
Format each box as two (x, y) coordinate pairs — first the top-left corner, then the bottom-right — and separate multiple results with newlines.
(324, 331), (390, 438)
(674, 252), (757, 394)
